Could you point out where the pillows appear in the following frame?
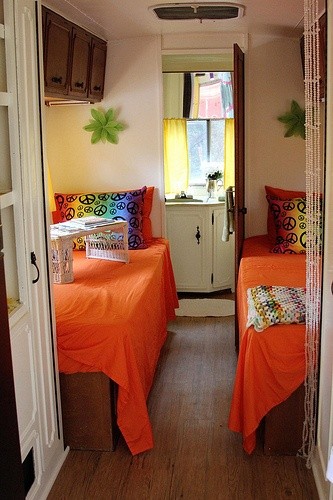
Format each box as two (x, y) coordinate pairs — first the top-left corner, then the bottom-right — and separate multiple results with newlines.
(52, 185), (154, 250)
(265, 186), (325, 254)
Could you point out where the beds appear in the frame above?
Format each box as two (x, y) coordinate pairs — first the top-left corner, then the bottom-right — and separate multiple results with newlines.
(50, 239), (169, 451)
(237, 235), (320, 456)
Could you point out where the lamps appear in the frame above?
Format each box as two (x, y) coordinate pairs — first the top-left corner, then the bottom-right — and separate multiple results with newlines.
(45, 100), (95, 106)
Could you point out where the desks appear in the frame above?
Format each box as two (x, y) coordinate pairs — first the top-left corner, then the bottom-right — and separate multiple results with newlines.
(50, 216), (130, 284)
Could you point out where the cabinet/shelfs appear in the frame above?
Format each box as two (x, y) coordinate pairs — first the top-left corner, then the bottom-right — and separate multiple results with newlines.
(299, 12), (327, 101)
(165, 204), (235, 293)
(42, 5), (108, 102)
